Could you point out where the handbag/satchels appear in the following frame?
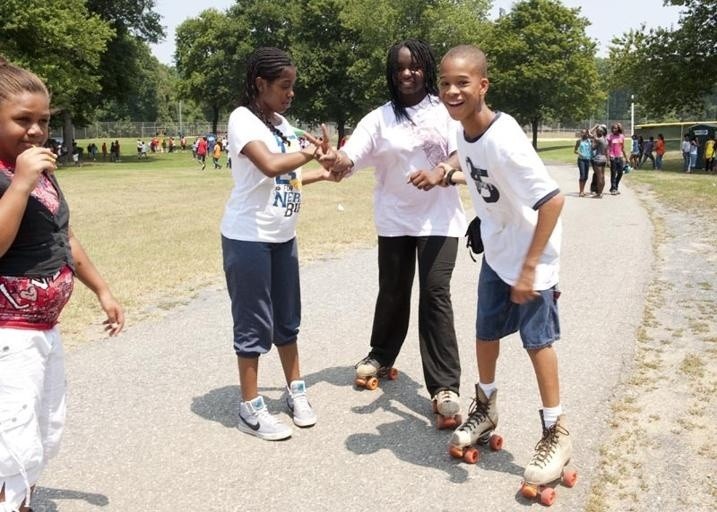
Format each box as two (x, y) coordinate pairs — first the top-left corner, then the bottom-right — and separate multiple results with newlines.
(465, 217), (484, 264)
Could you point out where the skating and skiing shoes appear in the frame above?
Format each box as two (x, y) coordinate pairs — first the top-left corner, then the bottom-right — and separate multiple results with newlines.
(431, 390), (462, 429)
(447, 383), (503, 464)
(522, 410), (577, 506)
(353, 354), (398, 391)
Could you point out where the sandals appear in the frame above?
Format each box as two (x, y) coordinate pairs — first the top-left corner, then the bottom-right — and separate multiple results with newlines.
(611, 188), (616, 194)
(616, 189), (619, 193)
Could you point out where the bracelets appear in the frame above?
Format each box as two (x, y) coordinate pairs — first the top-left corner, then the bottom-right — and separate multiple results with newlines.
(444, 168), (457, 186)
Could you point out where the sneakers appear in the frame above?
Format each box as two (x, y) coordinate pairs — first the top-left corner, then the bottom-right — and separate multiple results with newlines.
(236, 396), (292, 441)
(285, 380), (316, 428)
(202, 164), (206, 170)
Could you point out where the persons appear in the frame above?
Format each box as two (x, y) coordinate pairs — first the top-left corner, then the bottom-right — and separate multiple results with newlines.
(303, 38), (462, 428)
(575, 129), (592, 196)
(0, 58), (125, 512)
(196, 136), (207, 170)
(88, 144), (92, 158)
(607, 123), (630, 195)
(691, 135), (698, 171)
(110, 142), (116, 160)
(590, 124), (608, 194)
(589, 124), (607, 198)
(103, 143), (107, 161)
(138, 142), (147, 160)
(703, 134), (715, 172)
(208, 135), (215, 153)
(72, 150), (79, 163)
(114, 140), (120, 160)
(220, 48), (351, 441)
(652, 134), (665, 169)
(192, 142), (198, 159)
(682, 134), (690, 173)
(149, 133), (177, 153)
(709, 142), (717, 162)
(90, 143), (98, 160)
(640, 136), (655, 169)
(221, 136), (227, 150)
(433, 45), (578, 506)
(629, 134), (639, 169)
(181, 136), (186, 150)
(213, 141), (223, 169)
(137, 139), (142, 153)
